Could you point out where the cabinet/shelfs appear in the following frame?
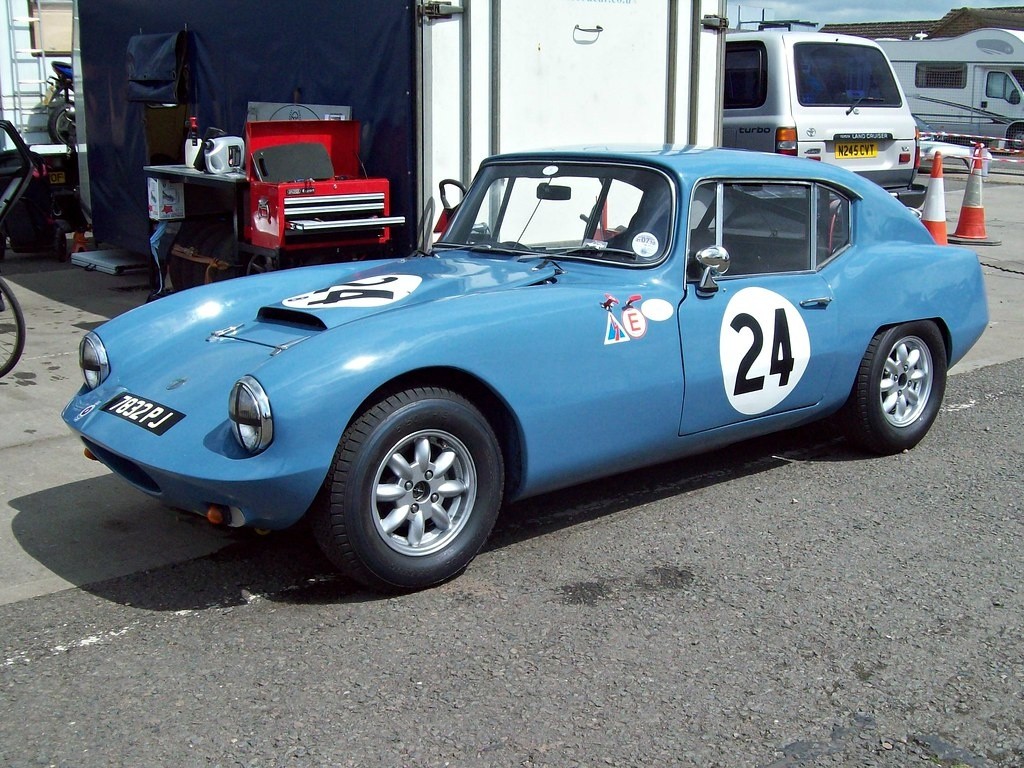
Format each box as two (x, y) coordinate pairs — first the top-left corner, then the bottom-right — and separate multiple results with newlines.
(143, 162), (248, 303)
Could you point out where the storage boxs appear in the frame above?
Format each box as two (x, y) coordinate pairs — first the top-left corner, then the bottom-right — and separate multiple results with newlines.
(244, 117), (406, 250)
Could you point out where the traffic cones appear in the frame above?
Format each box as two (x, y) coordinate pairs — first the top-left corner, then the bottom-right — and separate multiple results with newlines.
(947, 143), (1002, 246)
(920, 151), (948, 245)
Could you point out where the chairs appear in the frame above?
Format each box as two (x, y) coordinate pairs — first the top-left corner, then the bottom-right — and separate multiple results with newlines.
(620, 210), (669, 256)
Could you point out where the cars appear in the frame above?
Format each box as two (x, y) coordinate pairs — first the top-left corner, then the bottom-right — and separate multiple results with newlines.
(60, 147), (990, 593)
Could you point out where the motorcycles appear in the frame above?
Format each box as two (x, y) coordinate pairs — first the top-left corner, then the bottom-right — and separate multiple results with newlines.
(40, 60), (77, 147)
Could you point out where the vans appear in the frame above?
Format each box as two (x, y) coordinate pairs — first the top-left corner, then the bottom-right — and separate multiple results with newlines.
(723, 20), (929, 209)
(874, 27), (1024, 154)
